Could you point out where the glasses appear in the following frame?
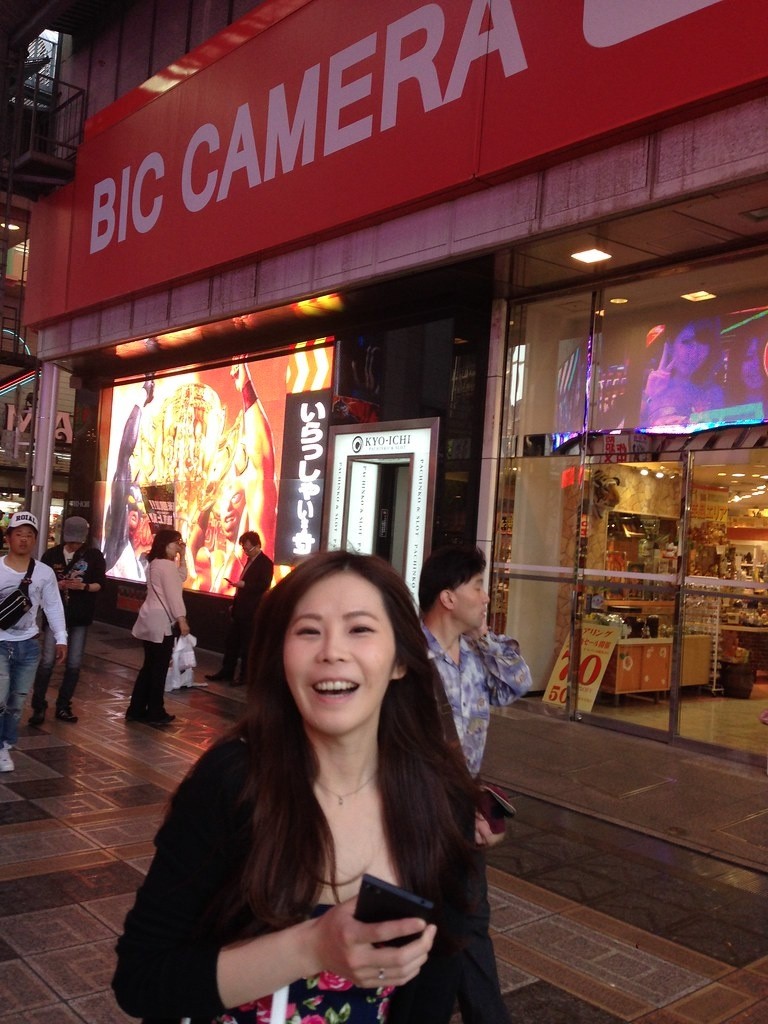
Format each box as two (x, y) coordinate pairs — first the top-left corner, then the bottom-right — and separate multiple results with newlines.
(244, 544), (258, 556)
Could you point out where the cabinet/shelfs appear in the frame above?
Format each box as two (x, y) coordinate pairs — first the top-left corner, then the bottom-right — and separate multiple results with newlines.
(672, 635), (712, 696)
(595, 638), (672, 707)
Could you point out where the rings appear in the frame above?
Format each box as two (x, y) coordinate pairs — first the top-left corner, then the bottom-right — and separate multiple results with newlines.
(377, 968), (385, 980)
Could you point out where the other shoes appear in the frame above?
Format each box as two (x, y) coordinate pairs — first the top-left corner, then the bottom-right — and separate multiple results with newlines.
(125, 707), (175, 724)
(28, 707), (45, 723)
(56, 707), (77, 721)
(231, 676), (248, 686)
(0, 740), (15, 772)
(205, 670), (234, 682)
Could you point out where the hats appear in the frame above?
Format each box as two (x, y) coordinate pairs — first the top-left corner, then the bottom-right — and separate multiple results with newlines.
(64, 516), (89, 543)
(8, 511), (39, 534)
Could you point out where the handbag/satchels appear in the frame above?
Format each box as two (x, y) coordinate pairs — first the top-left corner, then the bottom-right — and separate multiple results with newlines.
(0, 558), (35, 630)
(171, 620), (181, 636)
(164, 635), (197, 693)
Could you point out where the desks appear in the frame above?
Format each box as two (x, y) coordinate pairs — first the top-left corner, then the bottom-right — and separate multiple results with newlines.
(687, 621), (768, 632)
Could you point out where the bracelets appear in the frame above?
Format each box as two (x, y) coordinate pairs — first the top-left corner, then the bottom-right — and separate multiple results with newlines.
(84, 584), (89, 591)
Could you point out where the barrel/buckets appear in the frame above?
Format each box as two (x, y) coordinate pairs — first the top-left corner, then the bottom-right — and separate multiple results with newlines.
(721, 662), (757, 699)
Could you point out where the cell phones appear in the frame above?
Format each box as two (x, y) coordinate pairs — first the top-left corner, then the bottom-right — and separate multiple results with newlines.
(354, 873), (436, 949)
(65, 578), (73, 581)
(224, 578), (235, 585)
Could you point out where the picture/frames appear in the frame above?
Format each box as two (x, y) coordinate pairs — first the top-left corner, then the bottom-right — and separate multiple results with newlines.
(605, 551), (627, 600)
(626, 560), (646, 601)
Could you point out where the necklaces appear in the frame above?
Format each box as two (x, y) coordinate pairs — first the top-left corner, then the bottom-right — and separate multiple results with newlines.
(317, 775), (377, 806)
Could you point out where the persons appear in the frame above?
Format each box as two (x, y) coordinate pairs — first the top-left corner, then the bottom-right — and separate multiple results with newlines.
(124, 530), (189, 724)
(392, 544), (534, 1024)
(626, 562), (645, 599)
(27, 517), (106, 726)
(203, 532), (275, 688)
(0, 510), (5, 549)
(109, 550), (477, 1024)
(0, 512), (69, 771)
(606, 553), (627, 600)
